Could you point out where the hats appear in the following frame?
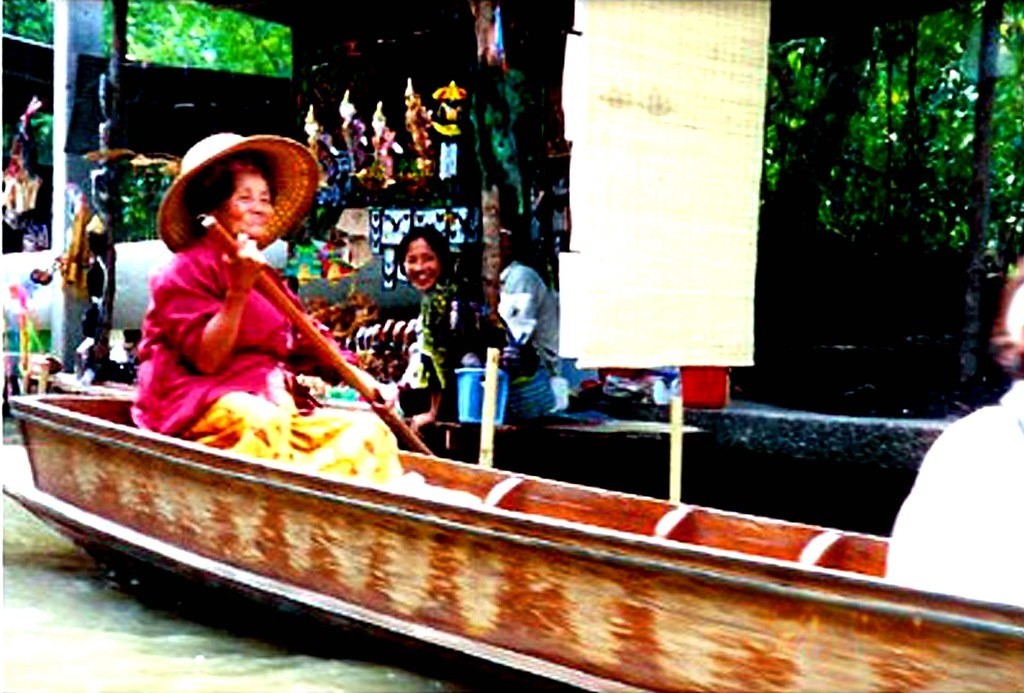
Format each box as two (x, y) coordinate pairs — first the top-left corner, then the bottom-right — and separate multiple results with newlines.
(157, 131), (318, 250)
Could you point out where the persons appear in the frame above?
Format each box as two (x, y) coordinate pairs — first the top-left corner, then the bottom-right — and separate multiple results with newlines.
(883, 257), (1024, 608)
(130, 131), (559, 485)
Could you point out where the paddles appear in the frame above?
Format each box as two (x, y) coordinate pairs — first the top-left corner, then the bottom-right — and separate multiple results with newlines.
(198, 213), (434, 456)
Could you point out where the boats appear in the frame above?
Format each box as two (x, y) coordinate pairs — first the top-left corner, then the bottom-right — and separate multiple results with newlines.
(0, 393), (1024, 693)
